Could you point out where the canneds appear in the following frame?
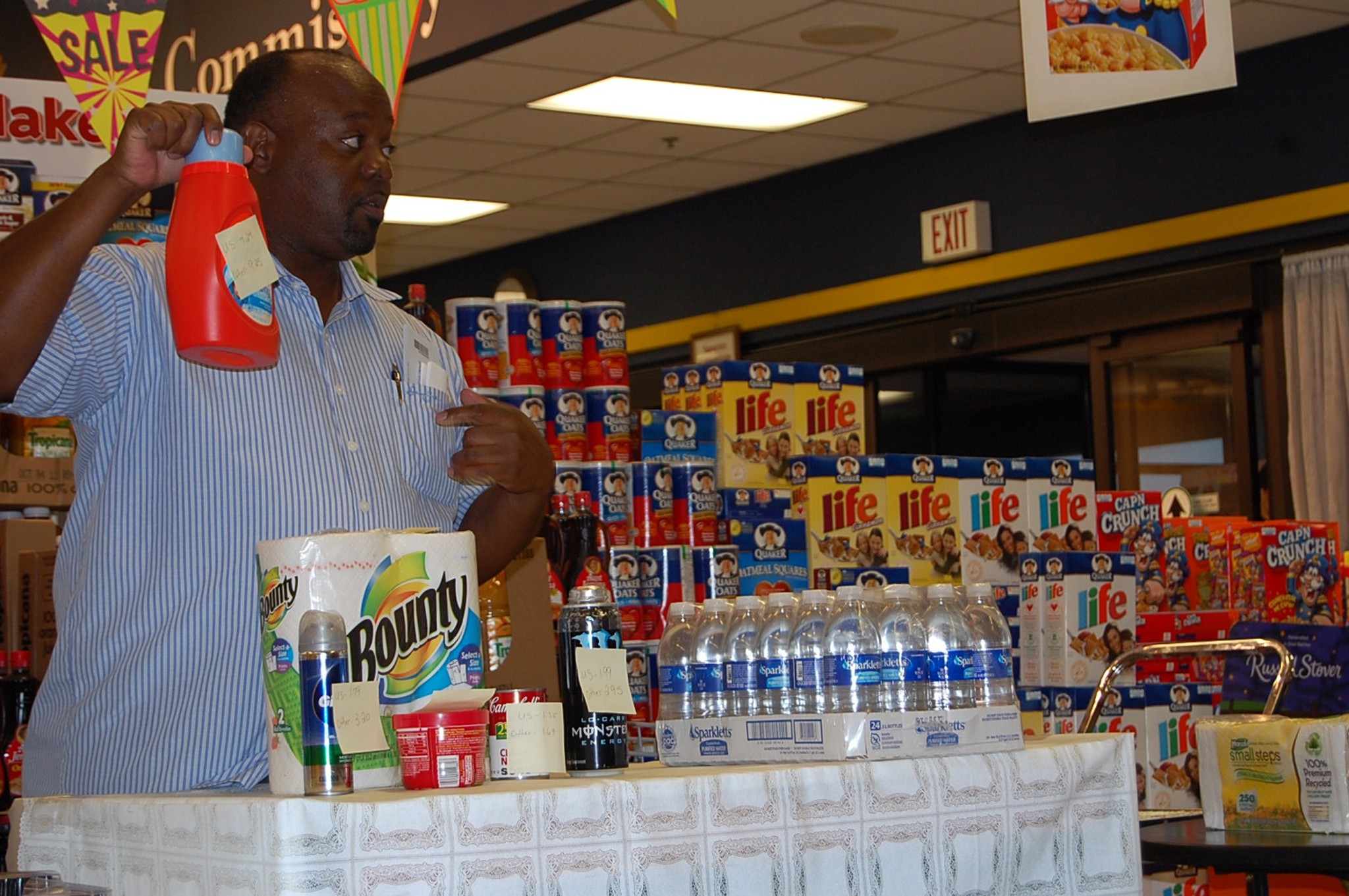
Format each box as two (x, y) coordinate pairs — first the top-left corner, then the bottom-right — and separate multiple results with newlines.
(447, 298), (742, 783)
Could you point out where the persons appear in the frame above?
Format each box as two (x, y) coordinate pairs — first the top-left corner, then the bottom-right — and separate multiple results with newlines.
(856, 458), (1096, 588)
(1041, 556), (1201, 809)
(484, 311), (622, 332)
(525, 395), (782, 678)
(0, 170), (69, 207)
(0, 47), (557, 798)
(765, 432), (860, 476)
(666, 364), (837, 388)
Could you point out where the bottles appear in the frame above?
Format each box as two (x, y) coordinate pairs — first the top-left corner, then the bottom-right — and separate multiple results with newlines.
(559, 586), (630, 775)
(299, 610), (355, 798)
(567, 490), (597, 590)
(404, 283), (441, 340)
(653, 580), (1016, 722)
(551, 492), (571, 588)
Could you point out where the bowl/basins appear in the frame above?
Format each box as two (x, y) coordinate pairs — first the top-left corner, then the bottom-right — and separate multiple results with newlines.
(393, 707), (491, 788)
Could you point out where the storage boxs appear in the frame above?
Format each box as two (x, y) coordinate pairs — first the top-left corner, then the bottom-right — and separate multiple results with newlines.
(656, 361), (1349, 811)
(0, 438), (76, 684)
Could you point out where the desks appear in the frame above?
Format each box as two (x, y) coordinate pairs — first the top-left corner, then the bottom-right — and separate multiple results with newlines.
(1141, 817), (1349, 896)
(20, 732), (1137, 896)
